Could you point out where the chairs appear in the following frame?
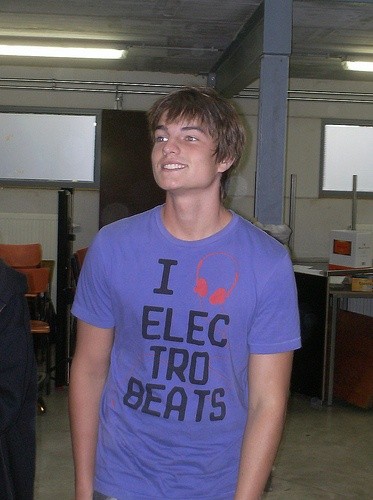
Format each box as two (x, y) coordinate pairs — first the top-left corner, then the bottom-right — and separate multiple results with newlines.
(0, 243), (88, 415)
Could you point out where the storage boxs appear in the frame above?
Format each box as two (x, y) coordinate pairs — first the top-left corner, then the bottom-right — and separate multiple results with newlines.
(328, 229), (373, 272)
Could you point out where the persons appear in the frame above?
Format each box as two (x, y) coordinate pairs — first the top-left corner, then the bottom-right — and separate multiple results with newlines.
(0, 259), (37, 498)
(67, 85), (302, 500)
